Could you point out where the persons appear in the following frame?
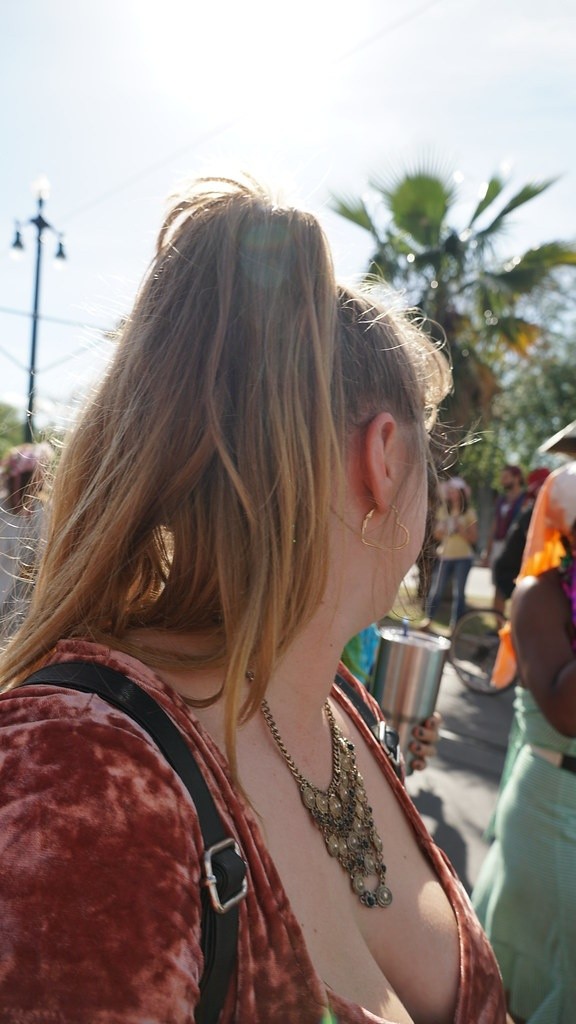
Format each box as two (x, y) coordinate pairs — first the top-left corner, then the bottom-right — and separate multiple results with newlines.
(0, 441), (55, 626)
(0, 173), (508, 1024)
(339, 457), (576, 1024)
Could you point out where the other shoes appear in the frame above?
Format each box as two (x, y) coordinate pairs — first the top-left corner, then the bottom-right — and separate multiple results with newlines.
(441, 629), (454, 639)
(415, 619), (432, 629)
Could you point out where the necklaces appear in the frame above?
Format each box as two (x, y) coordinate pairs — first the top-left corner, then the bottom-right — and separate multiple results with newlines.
(242, 670), (393, 908)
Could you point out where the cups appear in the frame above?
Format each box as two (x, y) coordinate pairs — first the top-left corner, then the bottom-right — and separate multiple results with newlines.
(369, 627), (449, 775)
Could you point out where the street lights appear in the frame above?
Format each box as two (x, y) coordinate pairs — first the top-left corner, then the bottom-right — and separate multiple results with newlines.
(11, 189), (68, 446)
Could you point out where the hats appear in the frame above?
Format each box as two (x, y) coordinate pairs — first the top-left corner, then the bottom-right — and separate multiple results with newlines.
(439, 476), (471, 501)
